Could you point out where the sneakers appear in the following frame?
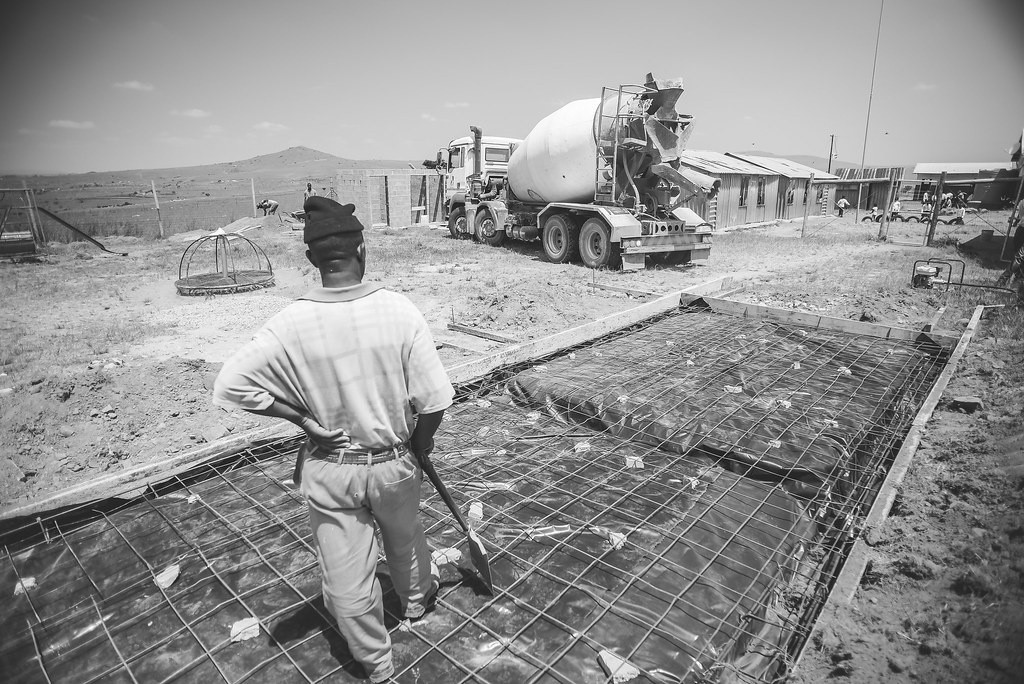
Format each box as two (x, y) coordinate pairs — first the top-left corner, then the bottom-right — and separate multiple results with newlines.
(363, 659), (395, 683)
(401, 562), (440, 618)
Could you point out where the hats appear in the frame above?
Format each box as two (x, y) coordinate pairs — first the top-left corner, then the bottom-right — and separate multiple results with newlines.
(303, 196), (364, 244)
(256, 203), (260, 209)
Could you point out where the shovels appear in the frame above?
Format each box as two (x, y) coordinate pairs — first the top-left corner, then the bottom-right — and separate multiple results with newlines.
(420, 450), (495, 598)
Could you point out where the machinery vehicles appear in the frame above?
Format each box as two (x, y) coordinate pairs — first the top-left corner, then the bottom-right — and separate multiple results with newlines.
(435, 71), (722, 274)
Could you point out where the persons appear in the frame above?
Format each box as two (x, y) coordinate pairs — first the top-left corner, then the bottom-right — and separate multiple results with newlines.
(304, 182), (318, 201)
(837, 194), (851, 217)
(213, 195), (455, 684)
(869, 203), (879, 221)
(921, 189), (965, 223)
(256, 198), (279, 217)
(888, 196), (900, 220)
(993, 198), (1024, 302)
